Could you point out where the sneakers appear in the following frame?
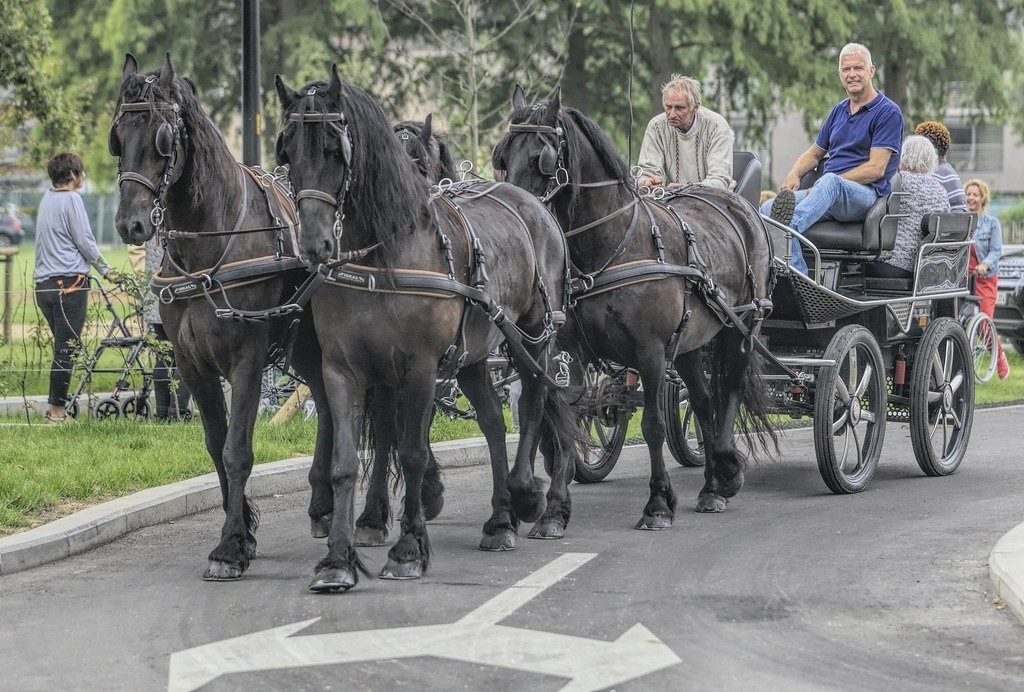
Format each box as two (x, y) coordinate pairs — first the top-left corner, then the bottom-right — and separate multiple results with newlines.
(997, 351), (1008, 378)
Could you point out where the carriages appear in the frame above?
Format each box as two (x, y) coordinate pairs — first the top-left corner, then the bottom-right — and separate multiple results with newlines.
(107, 50), (975, 593)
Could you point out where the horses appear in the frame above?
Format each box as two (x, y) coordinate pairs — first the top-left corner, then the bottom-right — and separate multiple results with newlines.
(113, 50), (791, 592)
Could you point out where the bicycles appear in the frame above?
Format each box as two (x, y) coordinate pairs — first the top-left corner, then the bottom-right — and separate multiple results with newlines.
(227, 354), (315, 425)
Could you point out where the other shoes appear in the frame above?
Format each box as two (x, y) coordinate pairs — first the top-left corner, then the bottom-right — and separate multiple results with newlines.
(770, 189), (795, 226)
(44, 409), (70, 423)
(153, 408), (191, 425)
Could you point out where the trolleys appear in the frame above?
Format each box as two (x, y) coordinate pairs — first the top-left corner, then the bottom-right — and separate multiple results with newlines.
(65, 274), (191, 426)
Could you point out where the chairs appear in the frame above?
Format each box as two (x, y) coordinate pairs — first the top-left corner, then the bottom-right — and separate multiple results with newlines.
(805, 174), (901, 250)
(734, 152), (762, 210)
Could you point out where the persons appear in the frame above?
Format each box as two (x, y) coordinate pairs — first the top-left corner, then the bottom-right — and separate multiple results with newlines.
(844, 134), (951, 278)
(32, 152), (118, 425)
(760, 43), (904, 277)
(127, 234), (191, 425)
(915, 122), (967, 212)
(963, 179), (1010, 380)
(638, 75), (737, 194)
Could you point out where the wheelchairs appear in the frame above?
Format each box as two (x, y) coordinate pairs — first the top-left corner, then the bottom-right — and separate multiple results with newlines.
(956, 267), (998, 384)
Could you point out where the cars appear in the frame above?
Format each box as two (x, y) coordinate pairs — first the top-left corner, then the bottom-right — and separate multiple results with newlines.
(992, 243), (1024, 354)
(1, 208), (25, 248)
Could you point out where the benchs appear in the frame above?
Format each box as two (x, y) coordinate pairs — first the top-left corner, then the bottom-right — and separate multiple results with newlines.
(866, 212), (978, 291)
